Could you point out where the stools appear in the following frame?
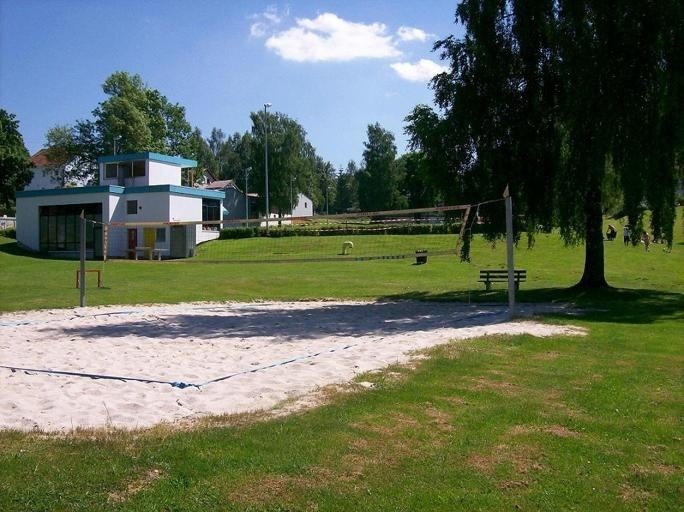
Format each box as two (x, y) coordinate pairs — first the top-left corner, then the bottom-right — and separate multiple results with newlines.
(76, 268), (104, 288)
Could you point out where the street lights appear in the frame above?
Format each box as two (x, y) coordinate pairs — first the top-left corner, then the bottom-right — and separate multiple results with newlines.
(325, 186), (332, 228)
(290, 175), (297, 227)
(241, 167), (252, 228)
(263, 102), (272, 234)
(113, 135), (122, 156)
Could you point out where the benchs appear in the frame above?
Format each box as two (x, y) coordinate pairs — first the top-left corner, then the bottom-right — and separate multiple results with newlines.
(477, 269), (527, 293)
